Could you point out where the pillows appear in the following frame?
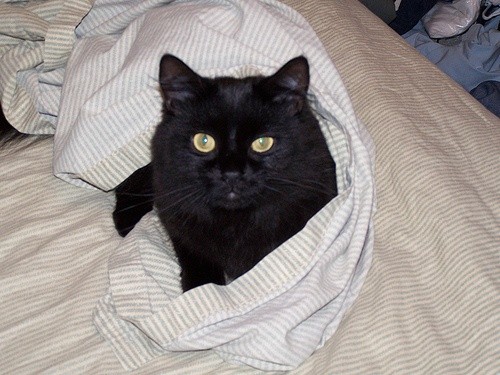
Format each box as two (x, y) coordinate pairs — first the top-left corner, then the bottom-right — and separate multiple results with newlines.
(393, 0), (481, 39)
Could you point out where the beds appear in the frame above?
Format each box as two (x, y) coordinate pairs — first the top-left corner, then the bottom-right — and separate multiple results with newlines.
(0, 0), (500, 375)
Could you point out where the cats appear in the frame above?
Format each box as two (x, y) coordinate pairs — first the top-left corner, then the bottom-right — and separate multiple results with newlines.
(111, 54), (339, 294)
